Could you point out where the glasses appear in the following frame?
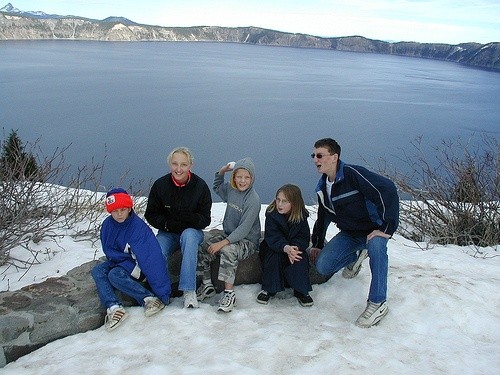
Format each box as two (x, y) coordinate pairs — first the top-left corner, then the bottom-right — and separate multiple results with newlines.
(310, 153), (333, 158)
(275, 199), (291, 205)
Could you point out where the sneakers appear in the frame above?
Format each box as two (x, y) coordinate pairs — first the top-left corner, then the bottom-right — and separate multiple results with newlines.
(183, 290), (199, 308)
(217, 290), (236, 313)
(355, 299), (390, 327)
(342, 249), (368, 278)
(196, 283), (216, 301)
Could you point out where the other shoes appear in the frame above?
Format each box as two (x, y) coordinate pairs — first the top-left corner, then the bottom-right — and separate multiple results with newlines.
(256, 289), (277, 305)
(294, 289), (314, 307)
(142, 296), (165, 317)
(104, 304), (128, 331)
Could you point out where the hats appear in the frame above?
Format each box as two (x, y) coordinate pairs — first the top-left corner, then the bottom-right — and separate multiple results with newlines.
(105, 188), (133, 213)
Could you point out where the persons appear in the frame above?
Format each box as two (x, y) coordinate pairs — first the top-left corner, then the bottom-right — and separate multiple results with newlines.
(309, 138), (400, 329)
(196, 158), (262, 313)
(145, 147), (212, 309)
(256, 183), (315, 307)
(91, 188), (171, 331)
